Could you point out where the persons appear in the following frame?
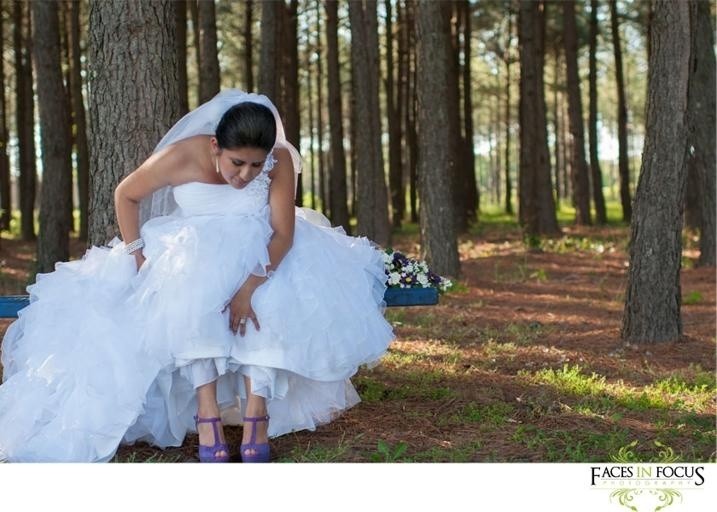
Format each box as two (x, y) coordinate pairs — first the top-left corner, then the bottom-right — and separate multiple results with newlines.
(1, 89), (394, 461)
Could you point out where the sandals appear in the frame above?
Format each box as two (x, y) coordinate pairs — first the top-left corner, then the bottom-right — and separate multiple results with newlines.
(240, 416), (271, 462)
(193, 412), (230, 462)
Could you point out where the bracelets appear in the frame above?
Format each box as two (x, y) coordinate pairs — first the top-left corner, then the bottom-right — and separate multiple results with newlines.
(125, 238), (144, 255)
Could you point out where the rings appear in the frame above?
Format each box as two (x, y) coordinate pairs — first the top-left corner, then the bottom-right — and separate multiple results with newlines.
(239, 318), (245, 325)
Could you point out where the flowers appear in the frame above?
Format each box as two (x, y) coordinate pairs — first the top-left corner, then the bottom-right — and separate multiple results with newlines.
(383, 247), (453, 292)
